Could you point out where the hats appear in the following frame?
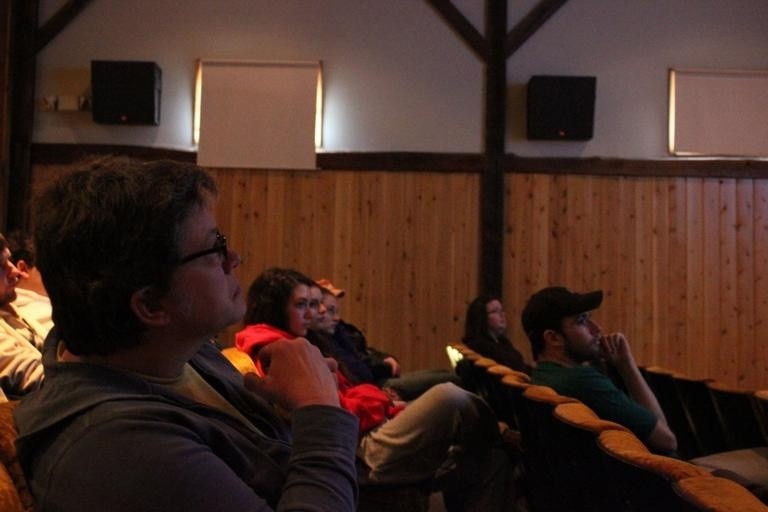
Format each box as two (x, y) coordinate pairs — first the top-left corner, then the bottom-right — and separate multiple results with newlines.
(520, 285), (604, 341)
(314, 278), (346, 298)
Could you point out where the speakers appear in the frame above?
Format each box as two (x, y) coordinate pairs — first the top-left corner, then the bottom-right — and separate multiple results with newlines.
(526, 74), (597, 141)
(90, 59), (162, 126)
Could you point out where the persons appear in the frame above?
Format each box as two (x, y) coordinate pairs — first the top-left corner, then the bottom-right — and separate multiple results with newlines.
(520, 286), (680, 457)
(319, 288), (507, 438)
(9, 235), (55, 342)
(13, 153), (361, 510)
(314, 278), (401, 378)
(235, 267), (510, 482)
(303, 278), (361, 395)
(462, 295), (535, 375)
(1, 232), (45, 401)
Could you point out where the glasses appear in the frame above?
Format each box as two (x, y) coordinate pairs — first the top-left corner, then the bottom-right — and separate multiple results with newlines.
(172, 233), (230, 269)
(486, 308), (507, 316)
